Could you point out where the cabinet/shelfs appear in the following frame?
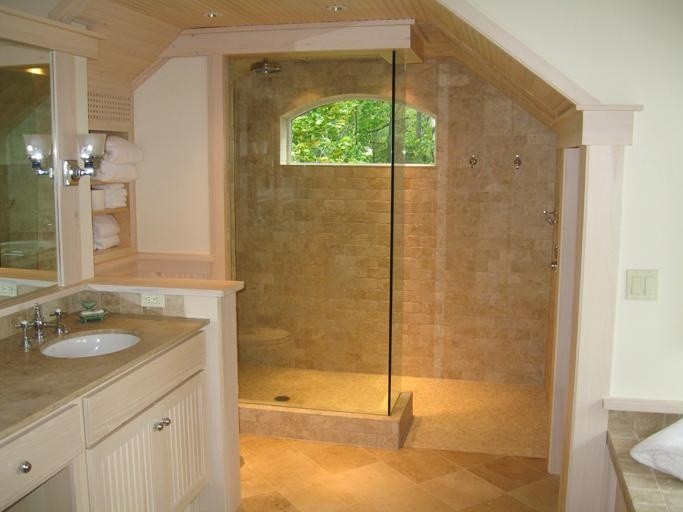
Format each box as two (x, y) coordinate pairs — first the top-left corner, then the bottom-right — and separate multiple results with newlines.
(0, 330), (212, 512)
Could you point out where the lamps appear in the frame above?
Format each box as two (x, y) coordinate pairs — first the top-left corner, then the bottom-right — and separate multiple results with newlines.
(23, 134), (53, 178)
(0, 38), (59, 307)
(62, 133), (108, 187)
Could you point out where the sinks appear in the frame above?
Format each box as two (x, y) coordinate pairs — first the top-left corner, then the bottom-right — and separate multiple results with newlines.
(38, 328), (142, 360)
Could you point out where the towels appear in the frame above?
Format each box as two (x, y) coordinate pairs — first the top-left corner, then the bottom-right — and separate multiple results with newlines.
(630, 416), (683, 483)
(91, 135), (144, 251)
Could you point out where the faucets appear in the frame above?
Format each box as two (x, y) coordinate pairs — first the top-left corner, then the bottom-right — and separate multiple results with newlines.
(33, 304), (64, 341)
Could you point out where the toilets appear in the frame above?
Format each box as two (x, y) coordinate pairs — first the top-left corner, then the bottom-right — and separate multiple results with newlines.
(238, 325), (295, 368)
